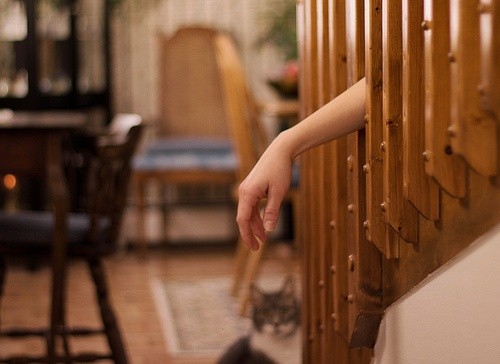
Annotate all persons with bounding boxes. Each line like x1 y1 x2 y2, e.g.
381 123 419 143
236 77 368 252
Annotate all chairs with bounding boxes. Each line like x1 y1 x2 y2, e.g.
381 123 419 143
1 112 144 364
124 26 257 248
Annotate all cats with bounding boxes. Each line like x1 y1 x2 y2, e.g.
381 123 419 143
217 272 302 364
